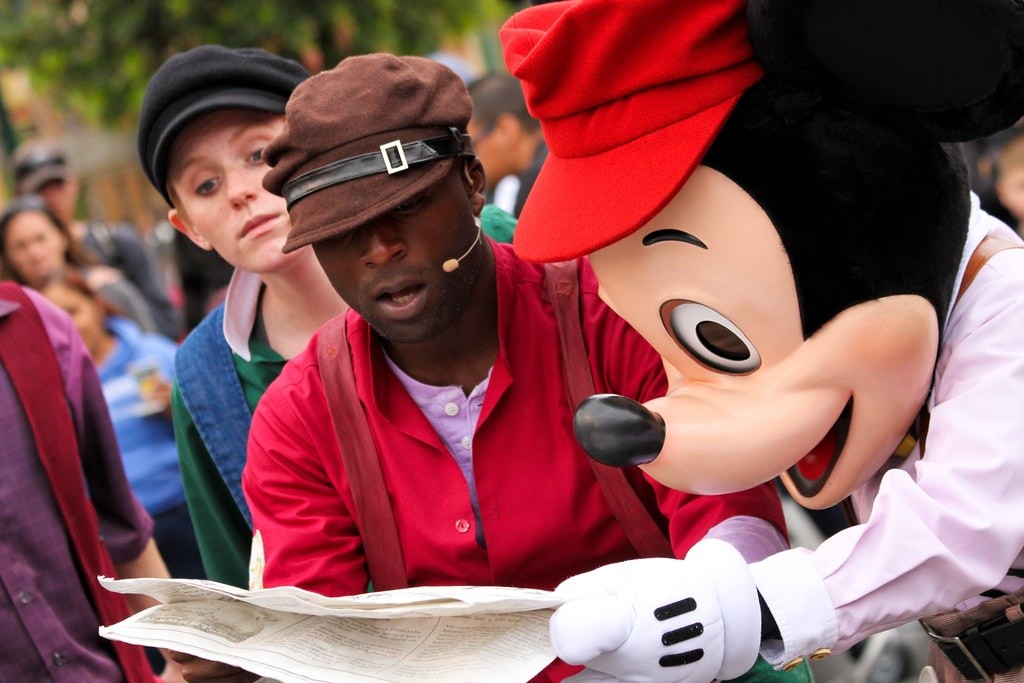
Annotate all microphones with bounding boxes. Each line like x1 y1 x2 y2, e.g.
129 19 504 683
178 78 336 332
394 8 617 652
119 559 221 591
442 228 481 273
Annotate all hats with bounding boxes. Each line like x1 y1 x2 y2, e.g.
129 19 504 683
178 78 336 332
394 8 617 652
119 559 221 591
137 43 313 208
262 52 476 253
14 145 70 194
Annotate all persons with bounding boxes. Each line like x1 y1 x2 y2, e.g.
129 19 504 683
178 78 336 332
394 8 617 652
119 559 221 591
0 44 1024 683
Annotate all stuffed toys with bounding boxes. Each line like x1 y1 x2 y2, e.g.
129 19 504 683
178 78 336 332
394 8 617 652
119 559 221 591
500 0 1024 683
